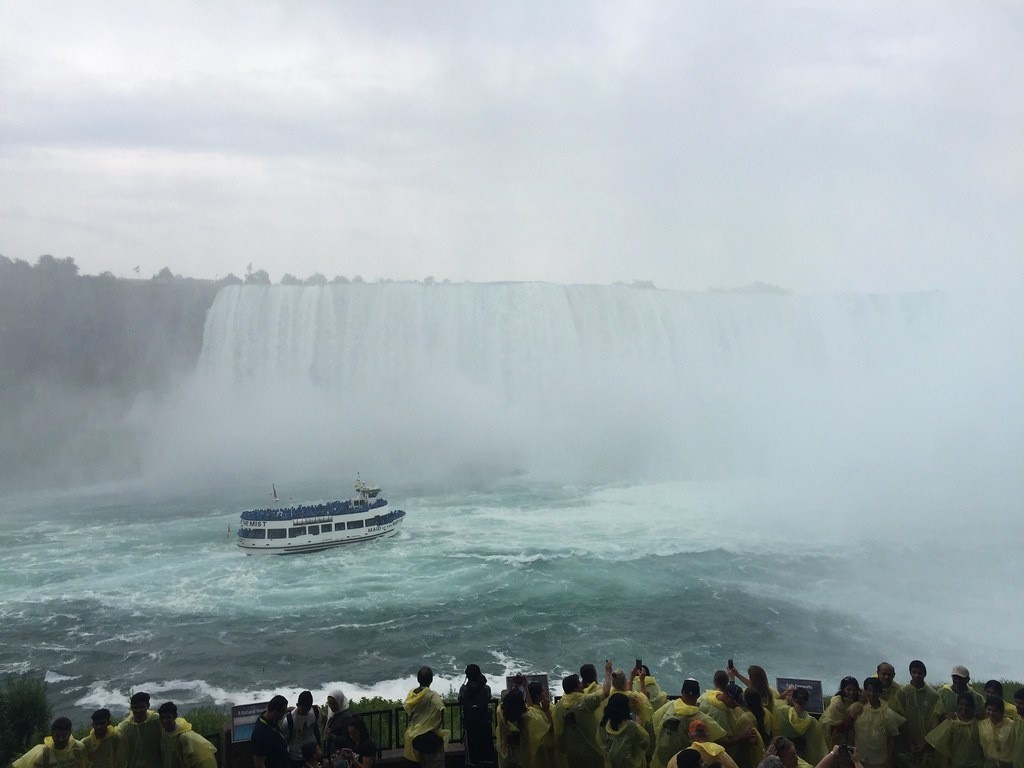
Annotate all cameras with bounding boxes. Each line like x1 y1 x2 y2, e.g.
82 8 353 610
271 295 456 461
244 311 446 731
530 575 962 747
513 675 524 686
839 744 854 757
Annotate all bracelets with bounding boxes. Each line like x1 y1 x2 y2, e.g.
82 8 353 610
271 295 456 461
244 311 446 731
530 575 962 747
830 751 835 756
730 680 735 681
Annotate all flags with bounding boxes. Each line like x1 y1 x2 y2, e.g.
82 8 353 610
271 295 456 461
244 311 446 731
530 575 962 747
272 484 279 502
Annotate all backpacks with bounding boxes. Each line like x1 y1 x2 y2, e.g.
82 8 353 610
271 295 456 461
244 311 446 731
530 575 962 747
287 706 321 744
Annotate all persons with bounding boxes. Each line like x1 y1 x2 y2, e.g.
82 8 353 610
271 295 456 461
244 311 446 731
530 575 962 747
237 497 406 539
11 659 1024 768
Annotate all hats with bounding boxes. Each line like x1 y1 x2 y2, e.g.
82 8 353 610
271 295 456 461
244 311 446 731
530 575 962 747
682 681 700 696
687 719 707 737
951 666 969 678
465 664 481 678
726 685 744 706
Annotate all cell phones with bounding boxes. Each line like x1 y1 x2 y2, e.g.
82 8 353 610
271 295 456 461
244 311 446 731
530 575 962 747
728 659 734 670
636 659 642 670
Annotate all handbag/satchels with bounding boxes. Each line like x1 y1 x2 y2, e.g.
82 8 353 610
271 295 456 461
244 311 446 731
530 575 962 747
412 730 444 753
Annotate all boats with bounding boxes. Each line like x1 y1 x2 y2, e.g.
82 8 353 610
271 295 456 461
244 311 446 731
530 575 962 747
236 480 406 556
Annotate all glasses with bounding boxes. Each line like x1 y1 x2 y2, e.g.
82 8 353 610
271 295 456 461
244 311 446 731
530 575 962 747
326 702 333 705
